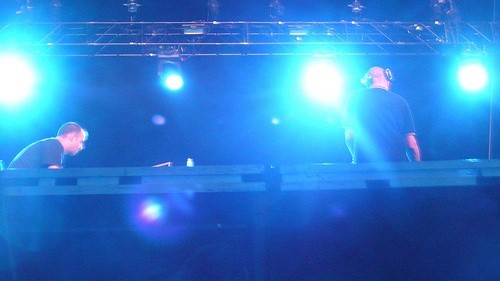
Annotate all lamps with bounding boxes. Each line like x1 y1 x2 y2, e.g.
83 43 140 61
157 58 186 94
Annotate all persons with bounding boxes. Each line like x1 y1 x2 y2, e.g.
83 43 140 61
344 66 422 161
6 121 88 170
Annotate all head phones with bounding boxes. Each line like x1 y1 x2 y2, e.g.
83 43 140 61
360 67 393 87
